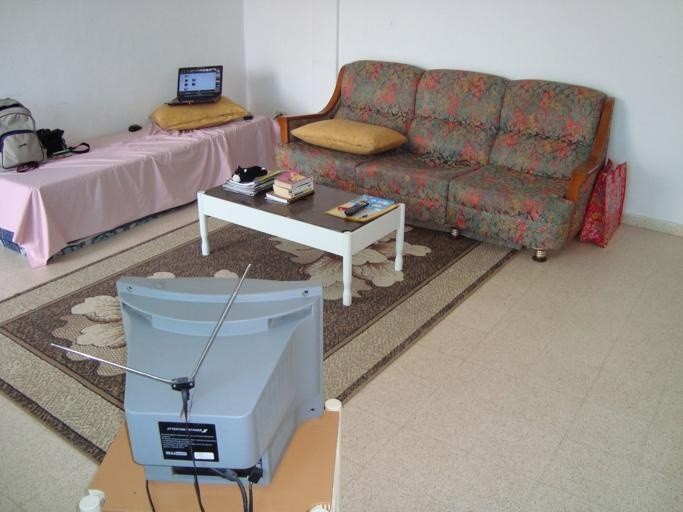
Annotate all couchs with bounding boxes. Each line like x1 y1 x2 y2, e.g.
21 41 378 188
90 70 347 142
272 60 615 262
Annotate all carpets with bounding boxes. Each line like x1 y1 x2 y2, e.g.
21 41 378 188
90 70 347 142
0 215 524 467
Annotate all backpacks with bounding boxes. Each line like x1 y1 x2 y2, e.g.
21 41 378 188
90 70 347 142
0 97 48 169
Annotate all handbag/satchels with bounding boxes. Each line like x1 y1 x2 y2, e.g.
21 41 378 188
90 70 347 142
37 129 90 156
579 158 626 247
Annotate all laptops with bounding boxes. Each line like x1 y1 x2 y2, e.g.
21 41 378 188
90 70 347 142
168 65 223 105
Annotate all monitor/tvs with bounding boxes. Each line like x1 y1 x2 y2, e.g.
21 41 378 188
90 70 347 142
116 277 325 487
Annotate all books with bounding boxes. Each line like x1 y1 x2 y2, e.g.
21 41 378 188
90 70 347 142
223 166 314 204
327 194 399 222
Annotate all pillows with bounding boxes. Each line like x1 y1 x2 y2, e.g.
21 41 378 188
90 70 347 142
149 95 251 132
289 118 409 156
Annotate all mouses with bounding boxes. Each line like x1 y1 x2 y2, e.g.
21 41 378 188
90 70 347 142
129 124 142 132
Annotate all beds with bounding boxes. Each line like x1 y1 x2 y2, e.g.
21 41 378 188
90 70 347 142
0 114 272 265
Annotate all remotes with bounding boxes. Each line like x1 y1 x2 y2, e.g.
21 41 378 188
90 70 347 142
345 201 369 216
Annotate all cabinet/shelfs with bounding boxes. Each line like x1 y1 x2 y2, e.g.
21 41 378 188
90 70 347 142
77 398 342 512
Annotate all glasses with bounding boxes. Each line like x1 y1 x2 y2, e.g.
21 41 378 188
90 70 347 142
17 160 40 172
